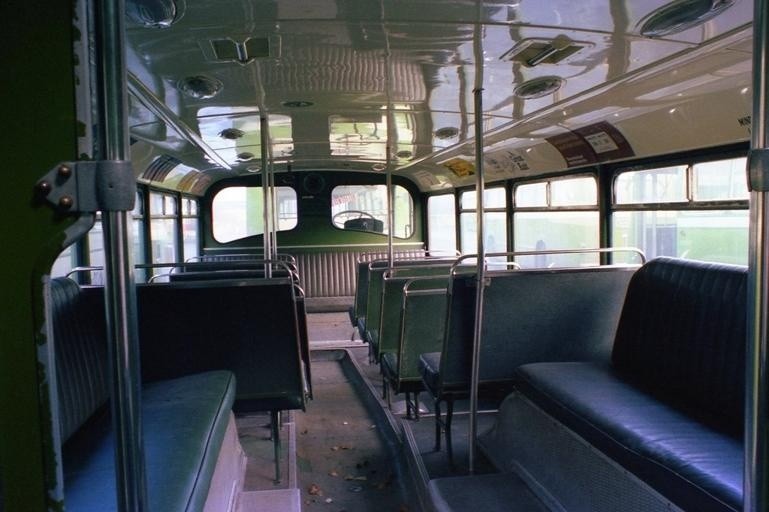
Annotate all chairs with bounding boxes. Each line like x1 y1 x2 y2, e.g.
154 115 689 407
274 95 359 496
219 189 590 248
382 276 450 422
360 257 488 341
49 276 238 512
65 261 310 484
293 283 313 400
370 263 522 375
418 246 647 470
516 255 751 511
169 262 298 273
343 219 382 232
348 250 461 342
186 254 296 265
147 269 301 287
